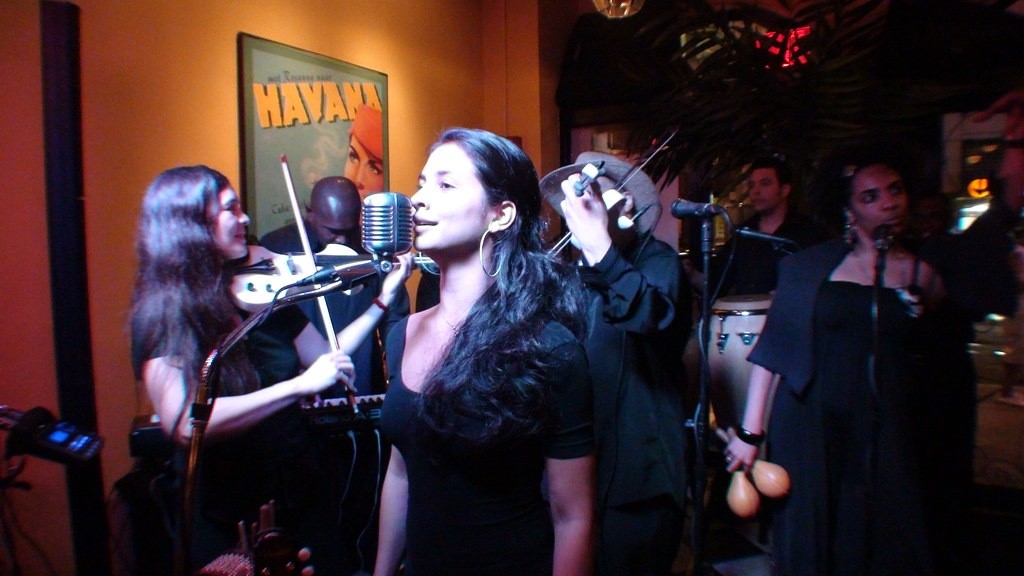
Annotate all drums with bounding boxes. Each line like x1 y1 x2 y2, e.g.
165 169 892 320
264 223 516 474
707 294 777 426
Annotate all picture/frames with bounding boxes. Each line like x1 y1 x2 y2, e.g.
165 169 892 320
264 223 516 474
235 31 390 245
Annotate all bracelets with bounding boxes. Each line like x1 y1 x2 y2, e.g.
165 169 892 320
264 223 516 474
734 425 766 447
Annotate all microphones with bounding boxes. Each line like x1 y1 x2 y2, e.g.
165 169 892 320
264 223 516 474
670 199 723 219
293 193 411 292
732 227 794 245
870 223 897 273
6 406 57 460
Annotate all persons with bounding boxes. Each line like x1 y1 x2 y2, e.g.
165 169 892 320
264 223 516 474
727 156 817 291
725 163 979 576
373 128 691 576
342 104 383 226
130 163 414 575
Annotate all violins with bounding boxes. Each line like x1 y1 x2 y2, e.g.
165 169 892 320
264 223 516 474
225 240 436 316
565 157 638 265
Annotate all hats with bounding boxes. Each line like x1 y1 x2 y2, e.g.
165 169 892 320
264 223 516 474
539 152 661 236
